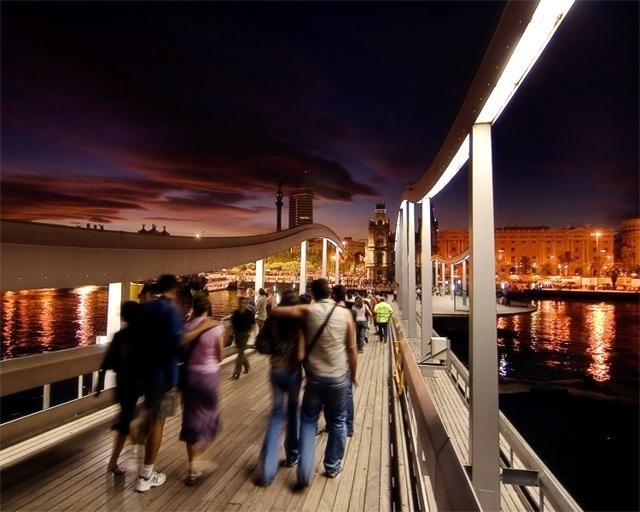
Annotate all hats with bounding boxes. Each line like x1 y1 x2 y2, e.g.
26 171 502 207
151 274 180 294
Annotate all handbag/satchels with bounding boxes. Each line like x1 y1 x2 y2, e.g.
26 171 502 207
99 322 136 372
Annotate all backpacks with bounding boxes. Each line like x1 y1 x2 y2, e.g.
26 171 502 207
252 313 286 356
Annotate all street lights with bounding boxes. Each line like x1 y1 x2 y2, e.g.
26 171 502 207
273 179 284 233
594 231 602 290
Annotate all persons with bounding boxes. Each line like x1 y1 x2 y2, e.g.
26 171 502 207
230 274 453 388
253 289 303 488
330 281 359 440
95 299 149 479
179 296 235 488
300 293 329 437
268 278 359 491
132 272 224 495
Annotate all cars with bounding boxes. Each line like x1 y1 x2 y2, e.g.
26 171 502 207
543 275 640 291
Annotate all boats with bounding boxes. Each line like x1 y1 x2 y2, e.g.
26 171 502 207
199 269 233 292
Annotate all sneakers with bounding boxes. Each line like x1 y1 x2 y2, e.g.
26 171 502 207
231 364 252 380
135 472 167 491
297 476 313 495
186 466 208 483
261 473 279 489
326 463 346 479
375 331 388 344
325 427 354 437
106 466 127 475
286 457 299 468
